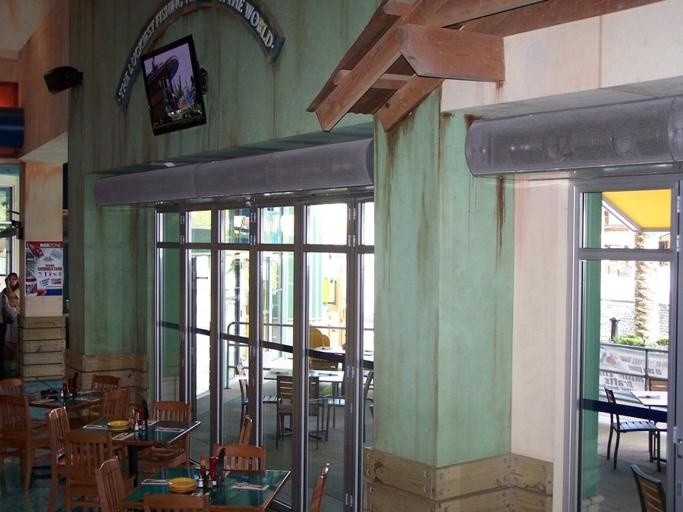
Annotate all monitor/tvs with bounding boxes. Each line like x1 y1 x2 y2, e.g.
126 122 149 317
139 35 206 136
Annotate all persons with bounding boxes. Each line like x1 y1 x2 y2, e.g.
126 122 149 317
0 273 21 378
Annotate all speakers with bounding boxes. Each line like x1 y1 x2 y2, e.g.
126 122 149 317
43 66 85 94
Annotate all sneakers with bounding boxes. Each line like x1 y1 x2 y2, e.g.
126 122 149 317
7 362 16 370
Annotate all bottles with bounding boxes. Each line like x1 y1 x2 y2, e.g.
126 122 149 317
196 448 226 488
59 376 78 399
128 406 147 432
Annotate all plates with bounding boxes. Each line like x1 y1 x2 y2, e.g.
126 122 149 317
106 420 129 427
167 477 197 492
107 427 128 432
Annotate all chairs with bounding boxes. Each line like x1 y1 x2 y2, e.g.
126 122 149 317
603 388 664 469
624 468 669 511
310 325 326 371
